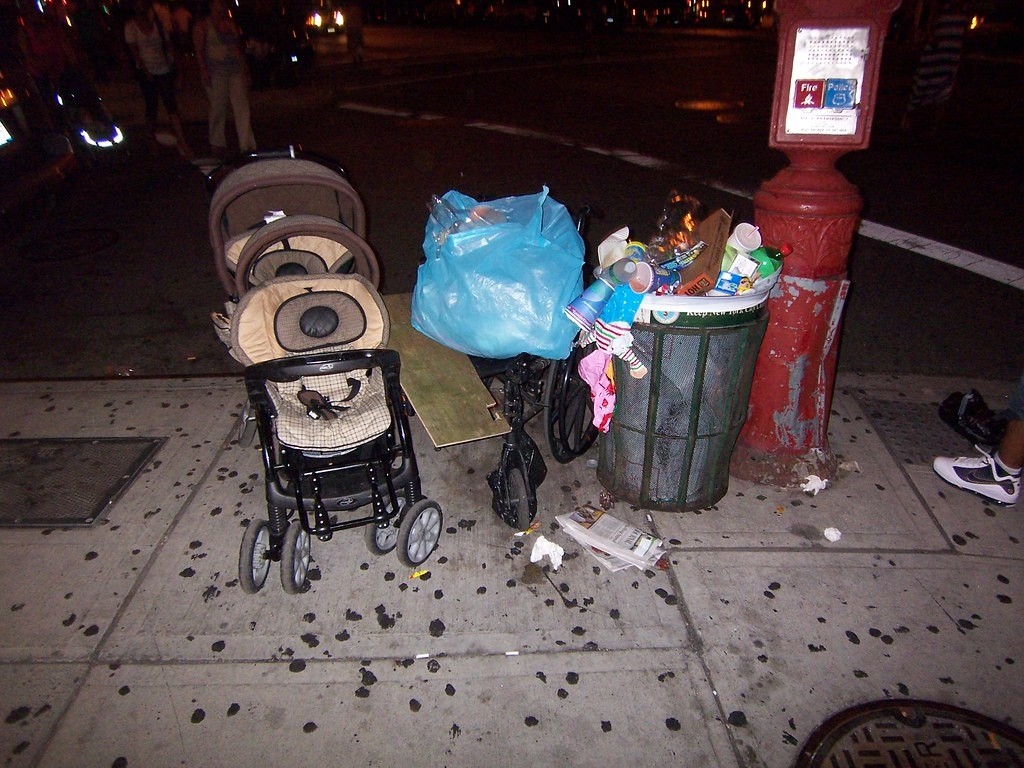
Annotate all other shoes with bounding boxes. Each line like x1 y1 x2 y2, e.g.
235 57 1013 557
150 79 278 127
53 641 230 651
212 146 229 158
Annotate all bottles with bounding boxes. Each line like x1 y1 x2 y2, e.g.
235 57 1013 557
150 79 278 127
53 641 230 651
600 259 635 285
747 244 792 282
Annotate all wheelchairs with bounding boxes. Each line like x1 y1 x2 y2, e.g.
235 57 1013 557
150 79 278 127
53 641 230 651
401 181 600 531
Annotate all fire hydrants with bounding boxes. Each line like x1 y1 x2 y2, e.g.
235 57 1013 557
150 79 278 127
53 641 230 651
727 0 897 485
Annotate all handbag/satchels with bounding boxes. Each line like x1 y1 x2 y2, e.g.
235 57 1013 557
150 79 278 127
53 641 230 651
243 46 266 80
160 73 184 108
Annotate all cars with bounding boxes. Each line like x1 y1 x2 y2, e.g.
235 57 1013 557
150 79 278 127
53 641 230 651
306 0 347 32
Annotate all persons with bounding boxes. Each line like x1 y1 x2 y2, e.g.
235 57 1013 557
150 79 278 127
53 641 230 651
933 377 1024 508
902 0 973 144
0 0 366 165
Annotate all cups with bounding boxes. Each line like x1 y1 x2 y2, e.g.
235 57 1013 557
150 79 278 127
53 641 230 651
564 278 614 332
625 242 645 265
728 223 763 252
628 262 682 293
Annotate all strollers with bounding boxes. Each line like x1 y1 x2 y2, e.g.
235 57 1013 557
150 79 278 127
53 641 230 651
203 146 443 595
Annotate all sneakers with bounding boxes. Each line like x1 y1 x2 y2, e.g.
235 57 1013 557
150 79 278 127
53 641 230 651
932 445 1020 507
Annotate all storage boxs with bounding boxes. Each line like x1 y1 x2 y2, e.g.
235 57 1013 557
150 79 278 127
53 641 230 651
676 207 735 295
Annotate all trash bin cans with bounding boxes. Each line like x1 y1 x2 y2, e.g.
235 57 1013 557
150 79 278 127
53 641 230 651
591 242 771 513
